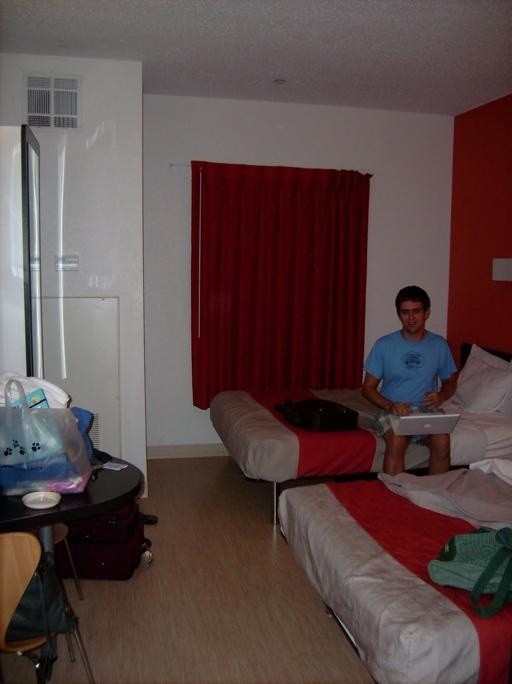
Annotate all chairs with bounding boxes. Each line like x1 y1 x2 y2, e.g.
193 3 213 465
27 522 84 600
0 531 96 684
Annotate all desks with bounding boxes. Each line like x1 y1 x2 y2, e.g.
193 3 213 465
0 456 141 660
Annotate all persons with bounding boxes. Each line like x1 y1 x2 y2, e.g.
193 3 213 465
363 283 464 475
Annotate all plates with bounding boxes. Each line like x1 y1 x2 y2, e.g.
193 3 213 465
21 492 63 510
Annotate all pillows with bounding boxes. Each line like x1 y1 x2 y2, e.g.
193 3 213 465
451 343 512 414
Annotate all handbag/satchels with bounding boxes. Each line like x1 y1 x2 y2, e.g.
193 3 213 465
0 379 93 497
277 397 360 430
427 526 511 617
6 549 79 646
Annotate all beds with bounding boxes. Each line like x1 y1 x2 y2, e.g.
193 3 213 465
209 389 512 526
278 468 512 684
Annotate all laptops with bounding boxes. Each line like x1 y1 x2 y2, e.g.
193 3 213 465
388 412 460 436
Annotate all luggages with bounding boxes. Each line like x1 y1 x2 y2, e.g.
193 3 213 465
60 502 161 580
